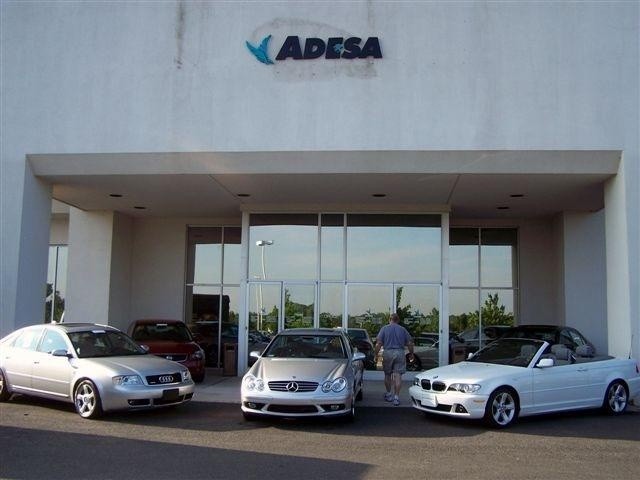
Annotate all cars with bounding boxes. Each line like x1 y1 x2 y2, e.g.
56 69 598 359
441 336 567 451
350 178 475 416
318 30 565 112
373 326 509 371
341 329 373 369
192 321 271 366
497 325 599 358
128 319 205 383
240 328 366 421
408 338 639 426
1 323 195 419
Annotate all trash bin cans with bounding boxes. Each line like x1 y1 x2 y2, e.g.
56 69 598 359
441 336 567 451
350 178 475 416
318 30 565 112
451 342 467 364
224 342 238 377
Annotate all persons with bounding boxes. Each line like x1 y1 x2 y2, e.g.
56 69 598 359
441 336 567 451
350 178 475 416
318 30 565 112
373 313 414 406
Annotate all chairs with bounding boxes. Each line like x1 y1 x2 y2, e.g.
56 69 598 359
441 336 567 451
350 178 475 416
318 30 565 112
318 343 339 357
520 345 536 359
575 344 592 361
555 349 572 365
550 343 566 354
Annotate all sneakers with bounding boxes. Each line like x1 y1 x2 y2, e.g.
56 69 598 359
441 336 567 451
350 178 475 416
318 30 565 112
384 393 399 405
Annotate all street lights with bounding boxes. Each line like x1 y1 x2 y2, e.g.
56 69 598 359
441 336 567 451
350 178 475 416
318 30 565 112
255 274 270 332
256 239 274 281
